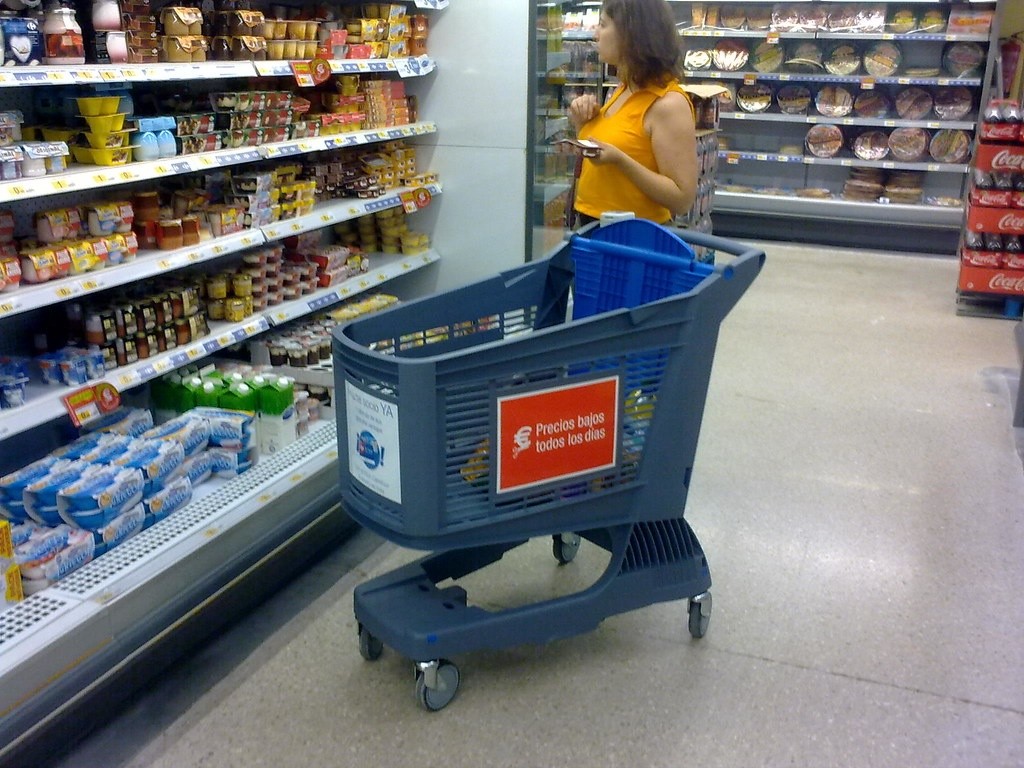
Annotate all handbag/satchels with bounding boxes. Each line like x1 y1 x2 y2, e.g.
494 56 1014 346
565 179 576 232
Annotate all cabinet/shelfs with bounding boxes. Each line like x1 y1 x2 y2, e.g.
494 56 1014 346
536 0 1024 321
0 0 443 768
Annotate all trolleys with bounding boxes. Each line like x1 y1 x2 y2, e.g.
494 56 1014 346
329 212 767 713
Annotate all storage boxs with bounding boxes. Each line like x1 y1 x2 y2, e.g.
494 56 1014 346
977 144 1024 172
980 121 1020 142
969 188 1012 207
1001 255 1024 270
1010 191 1024 209
962 246 1000 267
959 267 1023 294
298 79 440 203
967 192 1024 234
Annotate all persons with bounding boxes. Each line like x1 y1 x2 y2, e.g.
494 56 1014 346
570 0 697 227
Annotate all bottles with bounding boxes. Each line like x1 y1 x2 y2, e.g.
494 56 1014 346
622 389 657 463
956 99 1024 298
43 9 86 65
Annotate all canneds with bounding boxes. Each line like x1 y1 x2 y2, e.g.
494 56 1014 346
0 192 245 294
83 282 212 371
161 7 267 61
